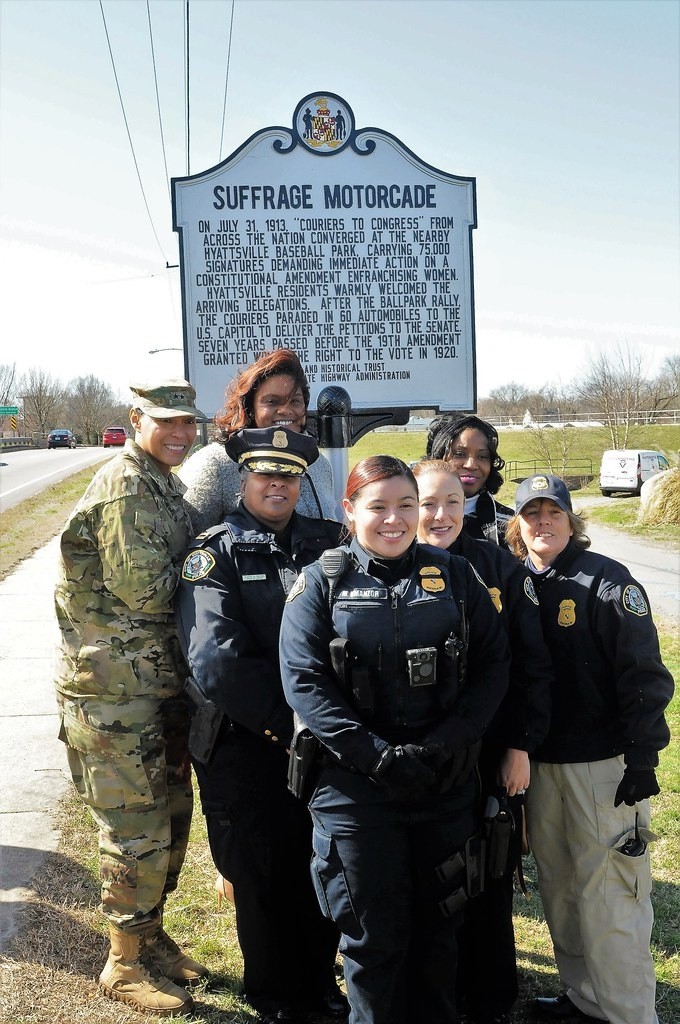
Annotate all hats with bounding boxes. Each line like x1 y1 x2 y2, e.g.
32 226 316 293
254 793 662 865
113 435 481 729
225 426 320 477
130 376 207 419
514 473 572 515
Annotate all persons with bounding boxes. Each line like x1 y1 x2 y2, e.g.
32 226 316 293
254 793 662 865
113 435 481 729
412 460 519 588
172 425 353 1024
278 456 555 1024
500 474 676 1024
425 412 518 550
176 352 338 909
55 375 206 1013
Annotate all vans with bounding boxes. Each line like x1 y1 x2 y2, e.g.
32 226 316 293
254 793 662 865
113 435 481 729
600 450 670 498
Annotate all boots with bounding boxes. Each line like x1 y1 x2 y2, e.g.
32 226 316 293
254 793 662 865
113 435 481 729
138 897 208 986
98 924 193 1018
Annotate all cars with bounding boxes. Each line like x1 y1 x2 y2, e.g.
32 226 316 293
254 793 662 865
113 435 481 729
47 430 77 449
103 427 126 448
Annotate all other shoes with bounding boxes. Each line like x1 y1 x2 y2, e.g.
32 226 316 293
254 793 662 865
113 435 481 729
296 969 349 1019
527 993 610 1024
246 990 308 1024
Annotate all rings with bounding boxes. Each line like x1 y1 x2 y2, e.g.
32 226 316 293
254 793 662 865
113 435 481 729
517 789 525 793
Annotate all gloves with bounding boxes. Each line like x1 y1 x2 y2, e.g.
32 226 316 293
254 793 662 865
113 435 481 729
614 768 660 808
372 741 446 807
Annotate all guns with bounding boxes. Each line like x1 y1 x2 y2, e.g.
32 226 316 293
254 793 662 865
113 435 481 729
182 676 225 727
